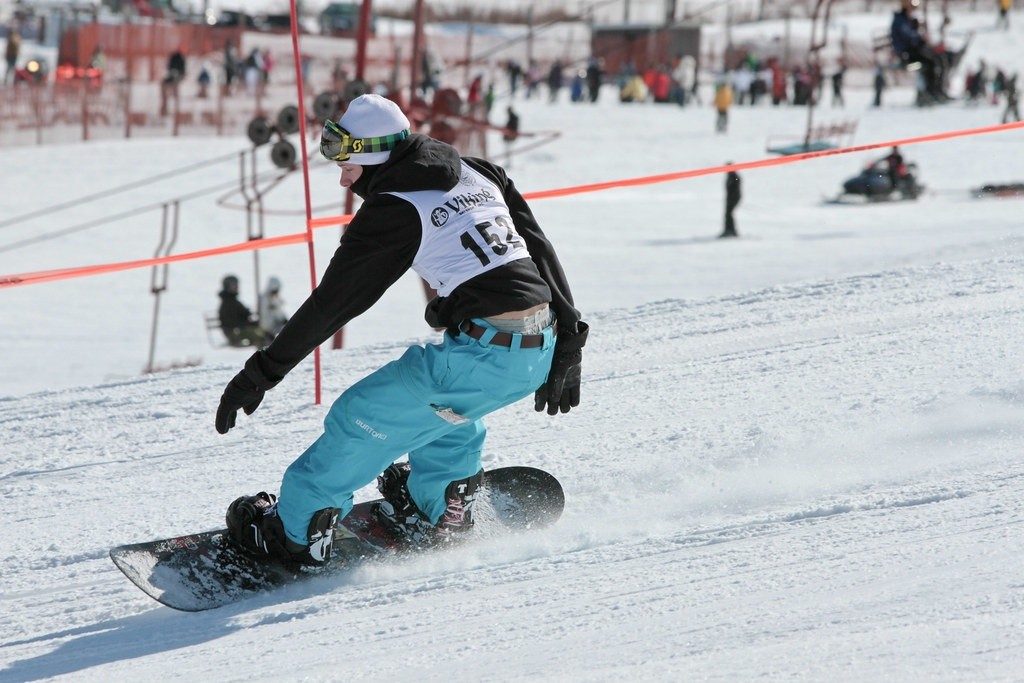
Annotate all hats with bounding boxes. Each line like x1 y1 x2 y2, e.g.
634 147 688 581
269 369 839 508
268 278 280 291
338 93 411 165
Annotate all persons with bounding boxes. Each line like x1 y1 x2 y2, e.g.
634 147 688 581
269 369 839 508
2 24 23 83
502 106 522 167
255 277 291 341
866 144 904 191
218 274 275 348
892 0 1023 127
215 93 589 569
85 33 563 123
717 162 742 237
571 39 891 133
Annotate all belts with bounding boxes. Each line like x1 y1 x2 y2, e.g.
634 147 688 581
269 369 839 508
454 316 558 349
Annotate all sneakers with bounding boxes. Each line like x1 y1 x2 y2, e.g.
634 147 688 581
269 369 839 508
378 461 475 540
225 496 333 572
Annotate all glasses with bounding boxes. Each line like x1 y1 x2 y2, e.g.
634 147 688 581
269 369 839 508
319 119 411 161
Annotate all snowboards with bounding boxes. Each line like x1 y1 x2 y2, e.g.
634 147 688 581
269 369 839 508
112 466 565 613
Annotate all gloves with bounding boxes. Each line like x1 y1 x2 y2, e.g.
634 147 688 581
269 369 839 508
215 348 284 434
534 320 590 415
